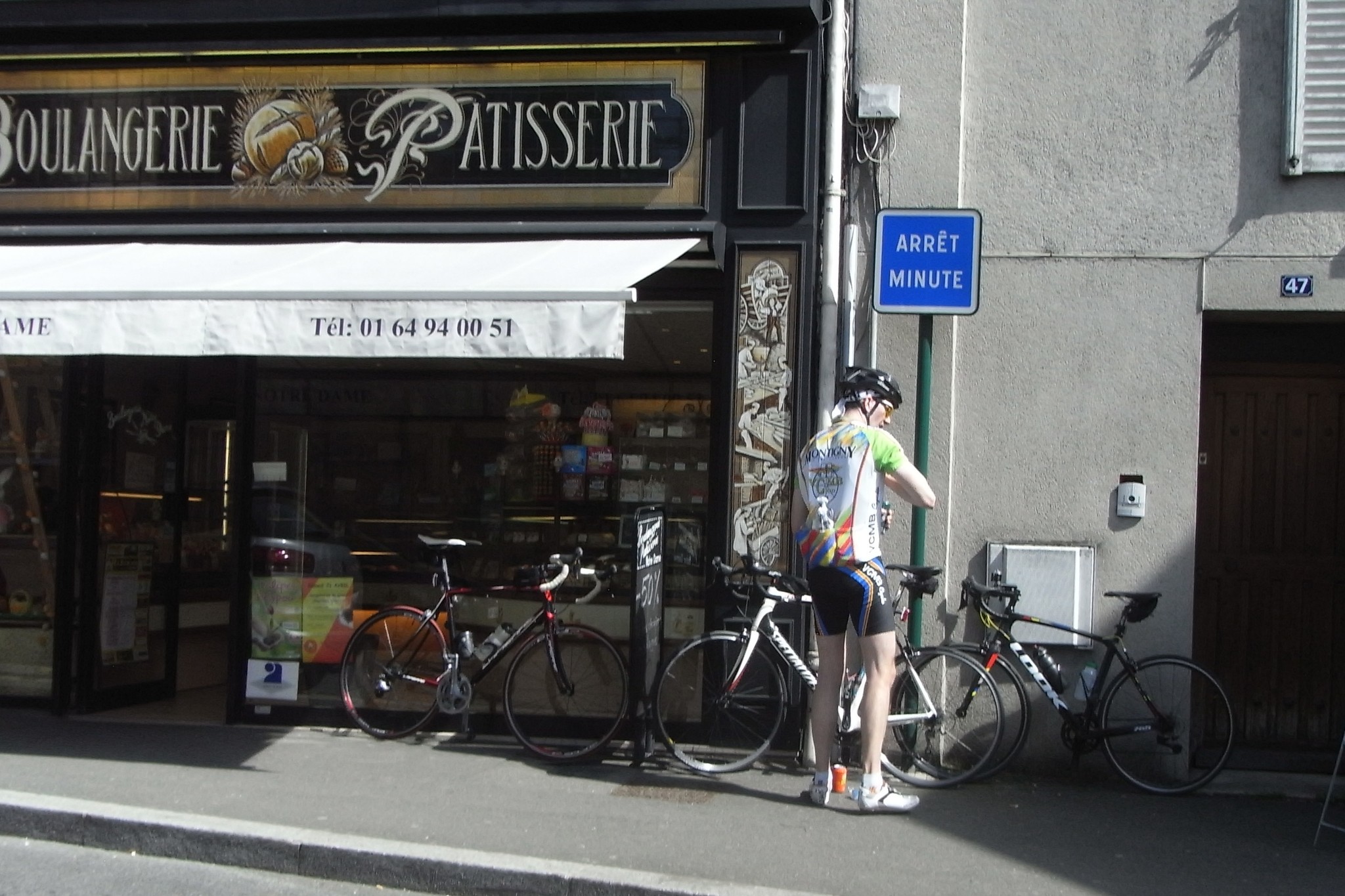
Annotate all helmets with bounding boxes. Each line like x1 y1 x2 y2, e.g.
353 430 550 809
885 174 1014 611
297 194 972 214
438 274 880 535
839 366 903 410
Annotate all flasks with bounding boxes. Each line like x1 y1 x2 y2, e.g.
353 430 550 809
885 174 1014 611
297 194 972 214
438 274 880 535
457 630 474 659
1032 643 1070 693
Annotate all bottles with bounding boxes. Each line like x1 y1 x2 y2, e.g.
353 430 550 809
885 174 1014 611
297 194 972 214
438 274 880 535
1073 661 1097 701
474 623 511 660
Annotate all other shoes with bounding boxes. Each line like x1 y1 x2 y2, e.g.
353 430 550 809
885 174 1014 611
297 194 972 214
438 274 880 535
857 782 919 813
808 777 833 805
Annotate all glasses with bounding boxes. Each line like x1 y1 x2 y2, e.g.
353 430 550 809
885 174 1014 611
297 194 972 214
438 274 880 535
883 403 893 417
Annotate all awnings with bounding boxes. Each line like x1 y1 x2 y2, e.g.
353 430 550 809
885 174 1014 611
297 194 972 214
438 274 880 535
0 219 727 361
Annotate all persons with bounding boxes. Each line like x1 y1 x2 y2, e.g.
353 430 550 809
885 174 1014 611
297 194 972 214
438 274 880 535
792 365 936 811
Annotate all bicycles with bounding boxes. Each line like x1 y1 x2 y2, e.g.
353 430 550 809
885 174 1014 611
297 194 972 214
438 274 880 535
650 555 1004 791
891 572 1234 797
341 532 629 764
